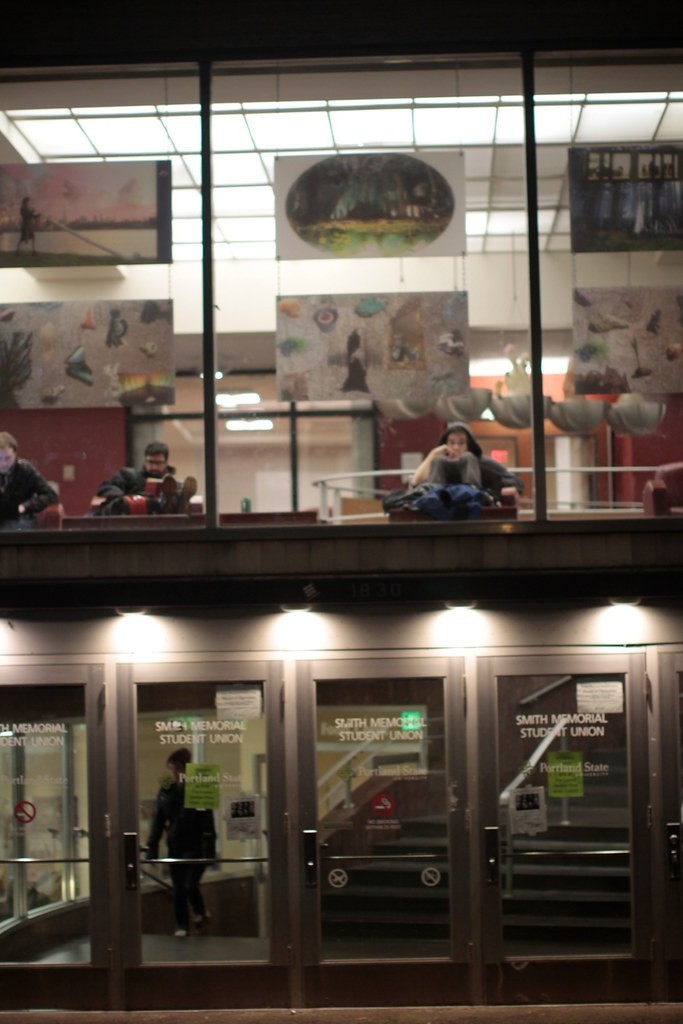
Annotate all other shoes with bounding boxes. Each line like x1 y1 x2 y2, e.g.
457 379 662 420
158 473 179 514
174 473 198 514
173 927 185 938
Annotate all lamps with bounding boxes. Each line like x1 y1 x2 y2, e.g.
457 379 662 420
372 299 667 435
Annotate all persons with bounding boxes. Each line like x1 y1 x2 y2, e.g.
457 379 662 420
145 749 217 940
91 442 193 515
0 432 59 530
408 422 524 506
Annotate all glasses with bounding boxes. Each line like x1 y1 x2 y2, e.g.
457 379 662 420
146 459 168 465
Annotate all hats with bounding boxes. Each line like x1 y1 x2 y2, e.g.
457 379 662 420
166 747 194 773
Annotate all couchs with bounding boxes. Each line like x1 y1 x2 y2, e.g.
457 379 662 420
642 460 683 518
32 503 67 532
88 495 205 516
388 485 519 522
